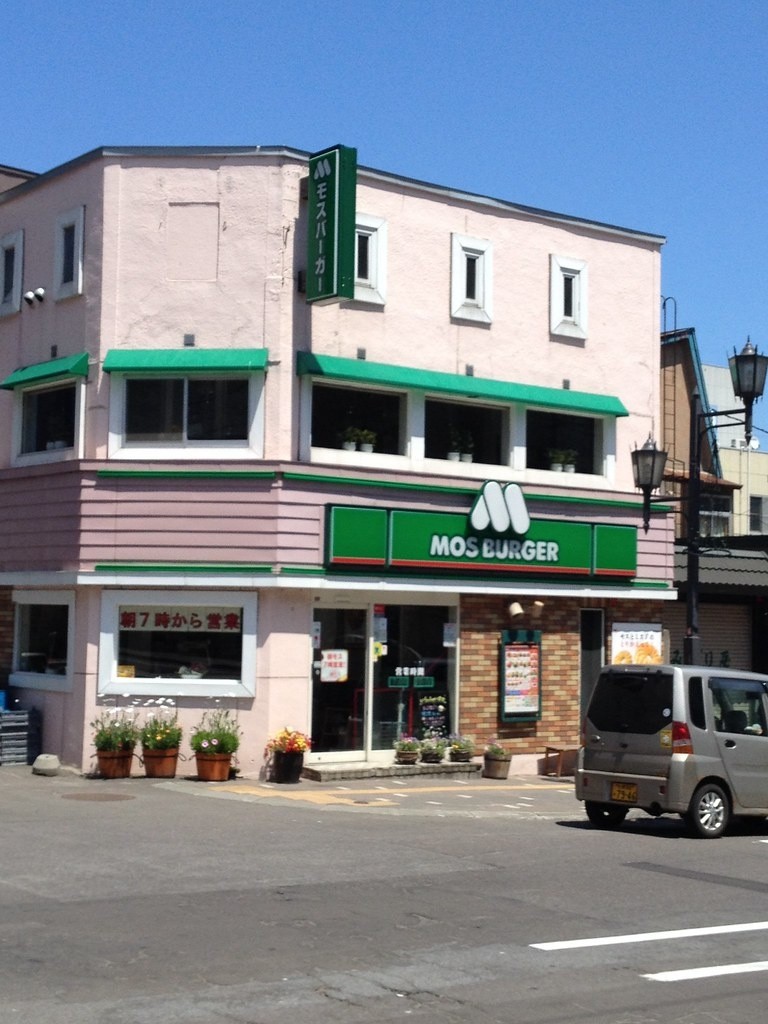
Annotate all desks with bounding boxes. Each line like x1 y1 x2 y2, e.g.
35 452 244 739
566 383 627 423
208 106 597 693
541 744 582 777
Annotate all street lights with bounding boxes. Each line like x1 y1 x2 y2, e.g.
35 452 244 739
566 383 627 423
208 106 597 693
631 334 767 665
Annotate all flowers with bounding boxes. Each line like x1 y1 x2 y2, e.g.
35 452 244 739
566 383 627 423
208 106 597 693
393 733 475 759
190 699 243 756
263 727 315 759
483 738 509 754
90 692 183 753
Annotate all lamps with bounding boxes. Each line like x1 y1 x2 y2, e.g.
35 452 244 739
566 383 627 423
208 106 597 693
505 600 544 621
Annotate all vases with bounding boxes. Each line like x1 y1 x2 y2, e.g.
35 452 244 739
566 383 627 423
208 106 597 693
142 748 179 777
196 752 231 781
421 751 441 762
484 755 512 779
397 750 418 764
97 750 133 778
450 751 469 762
273 751 303 784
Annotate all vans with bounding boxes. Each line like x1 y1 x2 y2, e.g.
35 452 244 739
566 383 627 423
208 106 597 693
574 662 768 840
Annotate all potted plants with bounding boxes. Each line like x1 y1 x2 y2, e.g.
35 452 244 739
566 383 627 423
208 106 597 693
46 427 70 450
340 426 377 452
445 433 474 463
549 448 579 472
